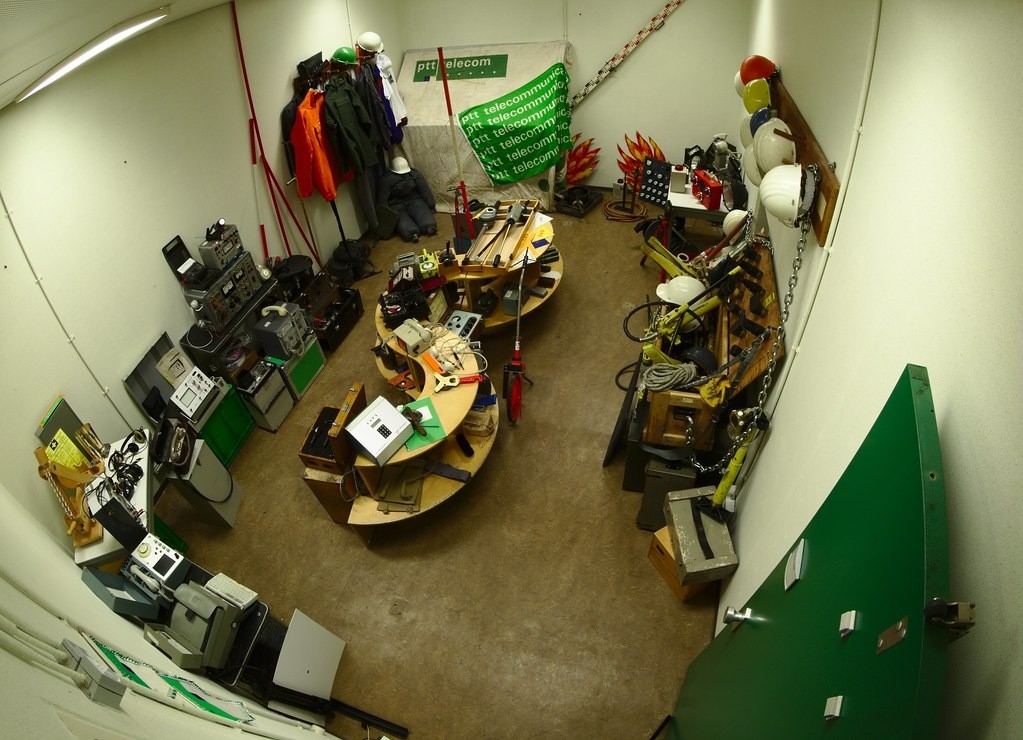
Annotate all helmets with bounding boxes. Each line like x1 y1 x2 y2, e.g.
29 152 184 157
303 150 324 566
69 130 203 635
739 114 754 148
757 164 803 227
330 47 359 65
732 71 745 96
723 210 749 245
388 157 412 174
357 31 385 53
754 117 796 172
749 106 771 137
722 178 748 212
742 142 767 187
655 277 707 311
684 345 720 376
741 78 771 113
740 55 775 85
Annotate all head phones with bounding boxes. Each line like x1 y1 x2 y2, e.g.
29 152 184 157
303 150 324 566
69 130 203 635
120 429 145 455
113 463 143 500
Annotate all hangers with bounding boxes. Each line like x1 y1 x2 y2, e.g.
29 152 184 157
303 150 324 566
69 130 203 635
290 49 379 92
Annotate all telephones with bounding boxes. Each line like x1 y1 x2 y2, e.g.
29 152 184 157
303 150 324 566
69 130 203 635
261 305 289 318
190 300 206 330
170 430 186 463
119 532 192 610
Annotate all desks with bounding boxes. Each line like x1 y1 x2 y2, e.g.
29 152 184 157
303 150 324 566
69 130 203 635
304 469 352 521
180 279 278 385
276 254 313 301
74 427 151 567
665 176 744 272
115 552 282 703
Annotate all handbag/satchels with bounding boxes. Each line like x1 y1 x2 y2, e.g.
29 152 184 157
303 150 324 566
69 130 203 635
332 239 372 265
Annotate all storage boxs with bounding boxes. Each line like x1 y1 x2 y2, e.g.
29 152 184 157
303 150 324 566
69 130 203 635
648 484 739 604
298 380 366 474
300 272 366 353
199 384 256 468
264 329 328 400
642 237 784 452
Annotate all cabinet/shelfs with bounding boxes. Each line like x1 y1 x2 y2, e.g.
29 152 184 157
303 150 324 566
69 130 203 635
347 199 560 529
